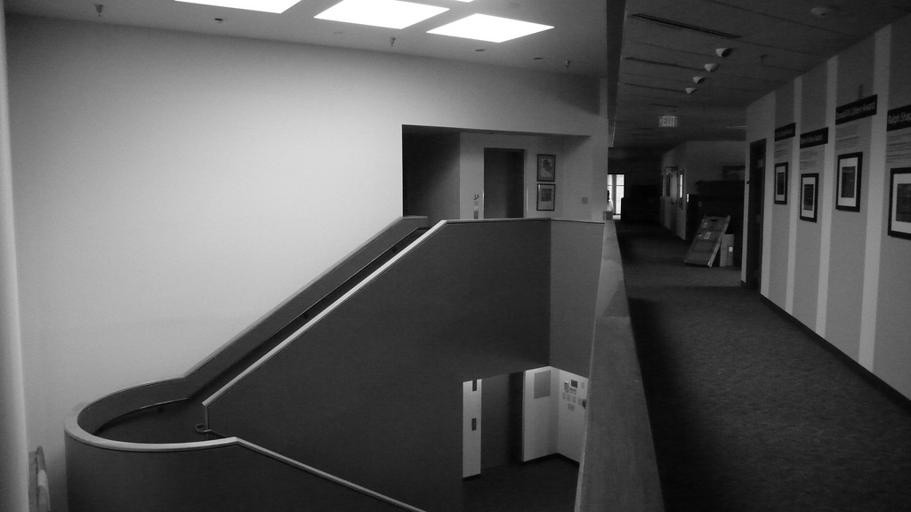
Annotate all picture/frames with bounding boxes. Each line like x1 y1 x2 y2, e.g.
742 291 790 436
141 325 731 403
888 166 911 241
537 154 555 181
774 162 788 204
836 152 863 212
536 183 555 211
800 173 817 223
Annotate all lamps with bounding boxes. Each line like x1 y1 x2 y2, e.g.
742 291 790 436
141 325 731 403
685 48 732 97
